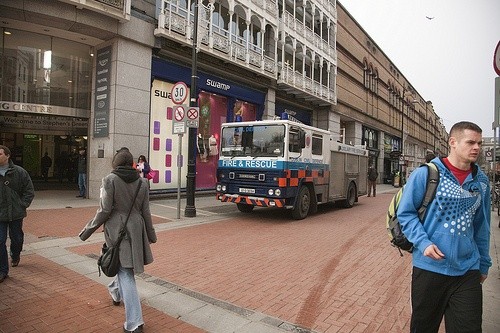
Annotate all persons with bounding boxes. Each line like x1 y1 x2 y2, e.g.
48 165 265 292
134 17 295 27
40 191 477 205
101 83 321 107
136 155 151 188
396 122 491 332
366 163 378 198
78 150 158 333
392 169 400 177
42 153 52 182
77 147 86 197
0 145 35 282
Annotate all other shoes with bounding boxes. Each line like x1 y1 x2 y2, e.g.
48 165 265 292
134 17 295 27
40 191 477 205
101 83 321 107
76 194 86 199
0 270 9 283
10 255 20 267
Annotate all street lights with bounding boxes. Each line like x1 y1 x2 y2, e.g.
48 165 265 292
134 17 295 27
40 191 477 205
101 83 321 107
399 100 420 189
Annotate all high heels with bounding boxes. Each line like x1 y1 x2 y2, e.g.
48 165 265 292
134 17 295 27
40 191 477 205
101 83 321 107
109 292 120 305
123 325 143 333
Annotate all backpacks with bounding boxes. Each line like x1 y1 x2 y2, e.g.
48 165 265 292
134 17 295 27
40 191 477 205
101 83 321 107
368 169 378 181
385 163 440 257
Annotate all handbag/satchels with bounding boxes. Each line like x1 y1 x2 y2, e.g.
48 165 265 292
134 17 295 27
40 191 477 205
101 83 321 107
144 167 156 179
97 243 120 278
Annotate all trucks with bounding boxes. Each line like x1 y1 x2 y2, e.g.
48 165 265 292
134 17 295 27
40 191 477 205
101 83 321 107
211 115 370 219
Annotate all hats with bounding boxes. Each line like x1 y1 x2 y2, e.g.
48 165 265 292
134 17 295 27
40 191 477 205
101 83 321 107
78 146 85 150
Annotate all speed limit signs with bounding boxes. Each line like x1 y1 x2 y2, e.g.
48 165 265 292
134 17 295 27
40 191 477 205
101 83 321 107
170 82 188 105
